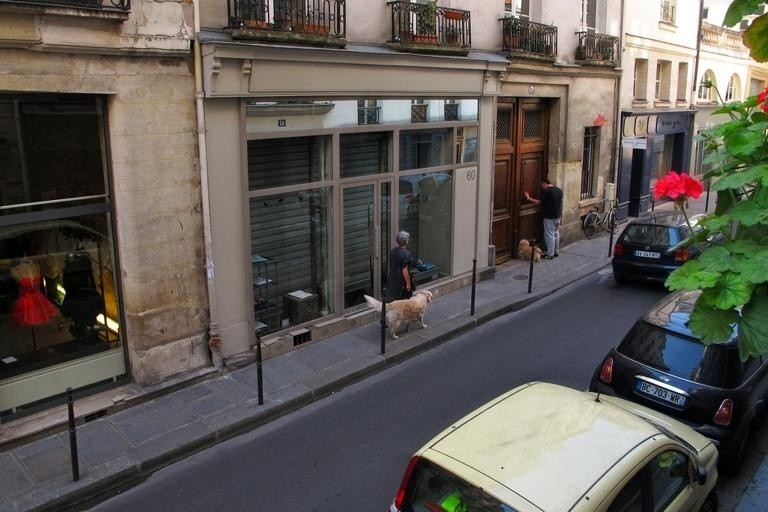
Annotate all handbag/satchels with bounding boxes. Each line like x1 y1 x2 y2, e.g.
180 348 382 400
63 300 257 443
402 274 416 299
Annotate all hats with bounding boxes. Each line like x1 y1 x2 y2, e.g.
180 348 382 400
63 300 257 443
397 231 410 241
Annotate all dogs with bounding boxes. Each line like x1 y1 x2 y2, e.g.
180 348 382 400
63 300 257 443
363 288 433 339
518 239 542 263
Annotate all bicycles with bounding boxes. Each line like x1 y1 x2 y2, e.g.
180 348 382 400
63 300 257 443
581 198 621 241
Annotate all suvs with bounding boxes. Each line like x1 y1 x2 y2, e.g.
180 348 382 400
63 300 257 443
612 208 726 290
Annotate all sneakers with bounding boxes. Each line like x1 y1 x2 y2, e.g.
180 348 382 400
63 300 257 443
540 253 559 259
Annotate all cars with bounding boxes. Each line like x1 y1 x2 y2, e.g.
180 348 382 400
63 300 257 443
390 377 721 512
589 288 767 473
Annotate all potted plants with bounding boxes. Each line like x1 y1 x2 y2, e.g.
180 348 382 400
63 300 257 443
439 24 463 45
403 0 438 45
273 0 293 31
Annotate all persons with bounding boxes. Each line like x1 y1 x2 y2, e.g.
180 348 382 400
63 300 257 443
11 257 58 325
524 177 563 259
388 231 412 302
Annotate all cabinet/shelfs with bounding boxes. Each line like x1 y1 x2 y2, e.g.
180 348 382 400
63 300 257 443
252 256 281 339
